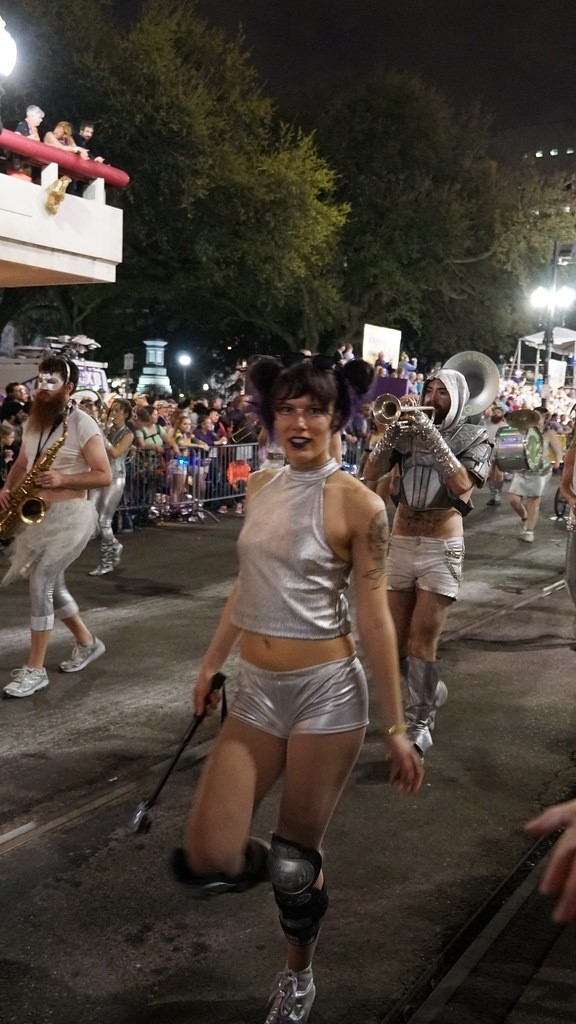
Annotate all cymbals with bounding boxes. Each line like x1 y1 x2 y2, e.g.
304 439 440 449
505 409 540 428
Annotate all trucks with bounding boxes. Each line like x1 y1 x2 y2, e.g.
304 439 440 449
0 336 109 404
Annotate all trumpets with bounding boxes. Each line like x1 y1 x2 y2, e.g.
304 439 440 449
373 393 438 432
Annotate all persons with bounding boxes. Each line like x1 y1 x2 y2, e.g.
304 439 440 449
560 421 576 606
88 398 135 574
363 368 495 758
525 799 576 919
508 408 561 542
166 351 422 1024
0 344 576 533
4 104 112 198
0 356 111 695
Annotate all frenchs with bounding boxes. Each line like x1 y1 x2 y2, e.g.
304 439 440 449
441 348 500 427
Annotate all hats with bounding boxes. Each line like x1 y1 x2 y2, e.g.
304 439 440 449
155 400 172 408
133 392 148 400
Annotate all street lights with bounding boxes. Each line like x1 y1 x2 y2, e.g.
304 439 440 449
528 239 576 408
179 355 191 397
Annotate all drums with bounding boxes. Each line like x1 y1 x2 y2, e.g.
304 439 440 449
494 426 544 472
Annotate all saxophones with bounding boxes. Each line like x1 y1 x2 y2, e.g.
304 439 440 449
0 411 69 540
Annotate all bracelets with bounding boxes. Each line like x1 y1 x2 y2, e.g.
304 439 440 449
382 724 410 736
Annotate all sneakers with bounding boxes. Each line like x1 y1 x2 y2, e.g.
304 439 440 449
3 665 50 697
263 960 316 1024
60 634 105 672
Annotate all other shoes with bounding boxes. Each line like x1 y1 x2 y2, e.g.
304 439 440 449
235 503 243 513
114 543 124 567
88 566 114 576
217 505 228 514
519 517 527 539
487 494 502 505
523 530 535 543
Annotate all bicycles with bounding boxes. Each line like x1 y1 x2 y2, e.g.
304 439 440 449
554 482 573 517
